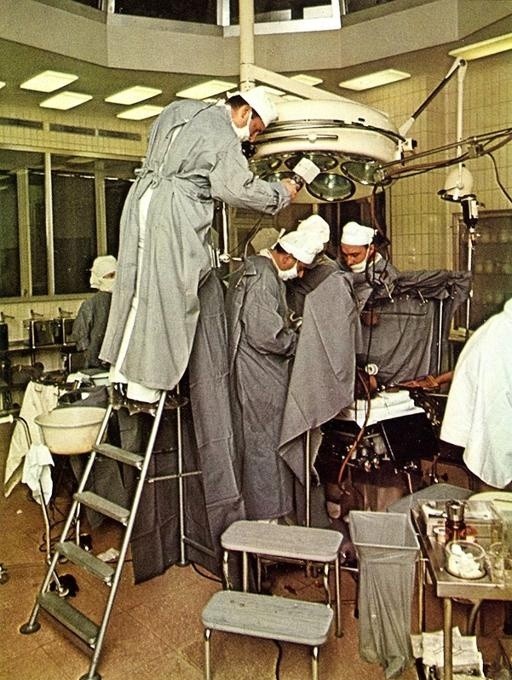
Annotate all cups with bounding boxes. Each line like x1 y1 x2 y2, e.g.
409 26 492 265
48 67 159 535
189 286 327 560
445 541 486 604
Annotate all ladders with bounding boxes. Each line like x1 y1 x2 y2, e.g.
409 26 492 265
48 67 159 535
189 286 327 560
20 391 217 680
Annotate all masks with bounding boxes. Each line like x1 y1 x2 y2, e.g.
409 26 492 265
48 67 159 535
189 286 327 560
232 119 251 141
349 258 368 274
277 262 298 281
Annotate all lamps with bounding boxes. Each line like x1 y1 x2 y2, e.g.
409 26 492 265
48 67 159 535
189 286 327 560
238 0 475 204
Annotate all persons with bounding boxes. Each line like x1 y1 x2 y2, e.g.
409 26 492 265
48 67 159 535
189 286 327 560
335 221 397 287
285 214 340 332
223 231 318 524
70 255 118 372
98 86 300 413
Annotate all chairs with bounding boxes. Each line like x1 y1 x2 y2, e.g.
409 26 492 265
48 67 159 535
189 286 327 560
201 520 344 680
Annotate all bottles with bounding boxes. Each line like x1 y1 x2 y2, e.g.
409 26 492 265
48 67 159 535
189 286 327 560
444 500 467 546
463 194 478 233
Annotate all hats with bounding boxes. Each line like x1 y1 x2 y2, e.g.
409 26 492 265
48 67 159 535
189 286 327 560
280 211 374 265
91 255 116 278
240 87 278 126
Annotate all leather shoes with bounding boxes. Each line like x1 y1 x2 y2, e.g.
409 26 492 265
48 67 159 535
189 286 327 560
127 391 189 410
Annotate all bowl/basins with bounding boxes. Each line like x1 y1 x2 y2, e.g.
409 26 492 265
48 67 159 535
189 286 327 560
34 406 107 455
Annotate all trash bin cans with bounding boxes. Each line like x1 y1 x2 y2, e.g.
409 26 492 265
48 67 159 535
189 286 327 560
349 510 420 666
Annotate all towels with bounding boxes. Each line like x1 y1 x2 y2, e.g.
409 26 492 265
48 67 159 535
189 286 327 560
21 444 55 505
